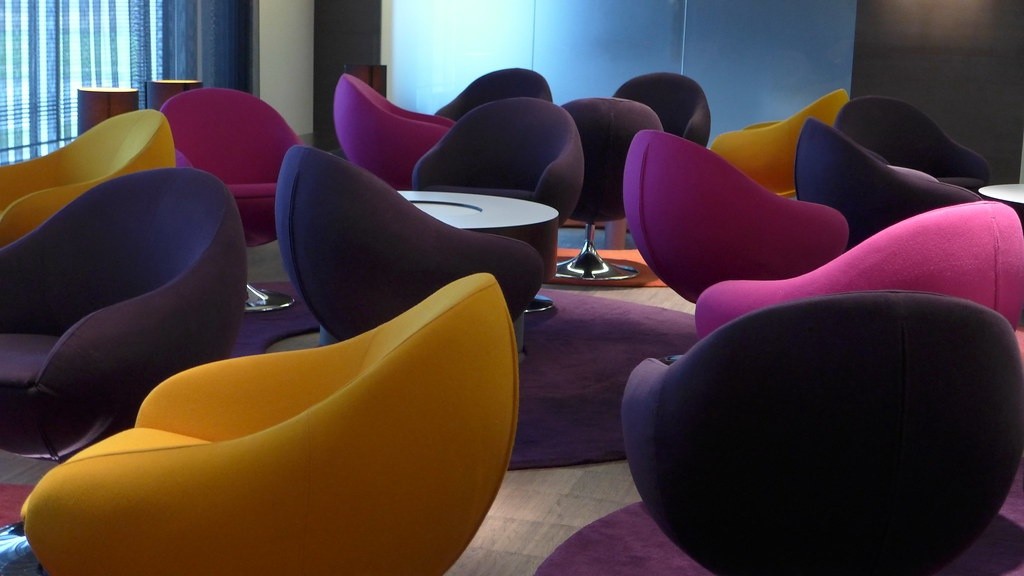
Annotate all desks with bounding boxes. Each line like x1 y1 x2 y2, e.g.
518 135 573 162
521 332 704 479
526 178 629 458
978 184 1024 238
396 190 559 362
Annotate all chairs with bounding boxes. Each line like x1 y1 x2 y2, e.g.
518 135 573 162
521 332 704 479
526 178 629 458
622 130 850 365
434 68 553 121
622 290 1024 576
333 74 456 190
794 117 982 251
275 145 545 341
412 97 584 226
556 97 664 280
710 88 849 199
21 272 519 576
0 168 246 462
0 109 176 249
696 200 1024 343
611 72 711 147
833 95 992 193
160 88 305 312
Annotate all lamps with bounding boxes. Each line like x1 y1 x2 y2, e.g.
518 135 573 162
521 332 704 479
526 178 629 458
77 86 138 138
344 64 387 99
148 80 204 111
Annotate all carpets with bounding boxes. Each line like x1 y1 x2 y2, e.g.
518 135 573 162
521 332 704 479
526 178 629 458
545 248 670 288
230 282 697 472
534 456 1024 576
